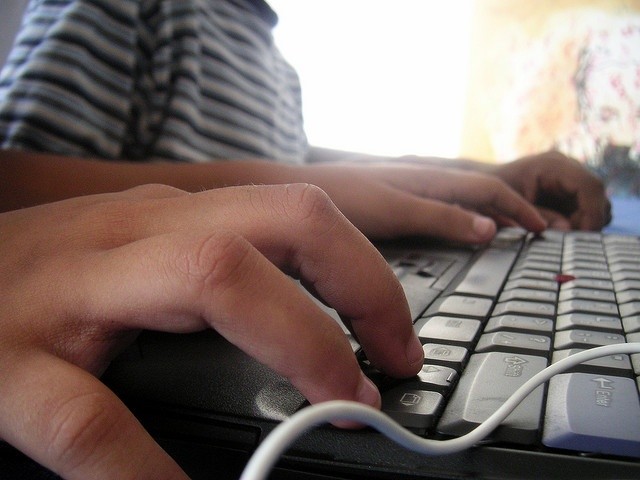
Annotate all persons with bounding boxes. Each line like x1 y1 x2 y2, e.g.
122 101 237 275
0 1 612 232
0 149 549 479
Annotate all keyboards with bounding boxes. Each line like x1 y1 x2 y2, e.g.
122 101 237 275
100 227 639 478
